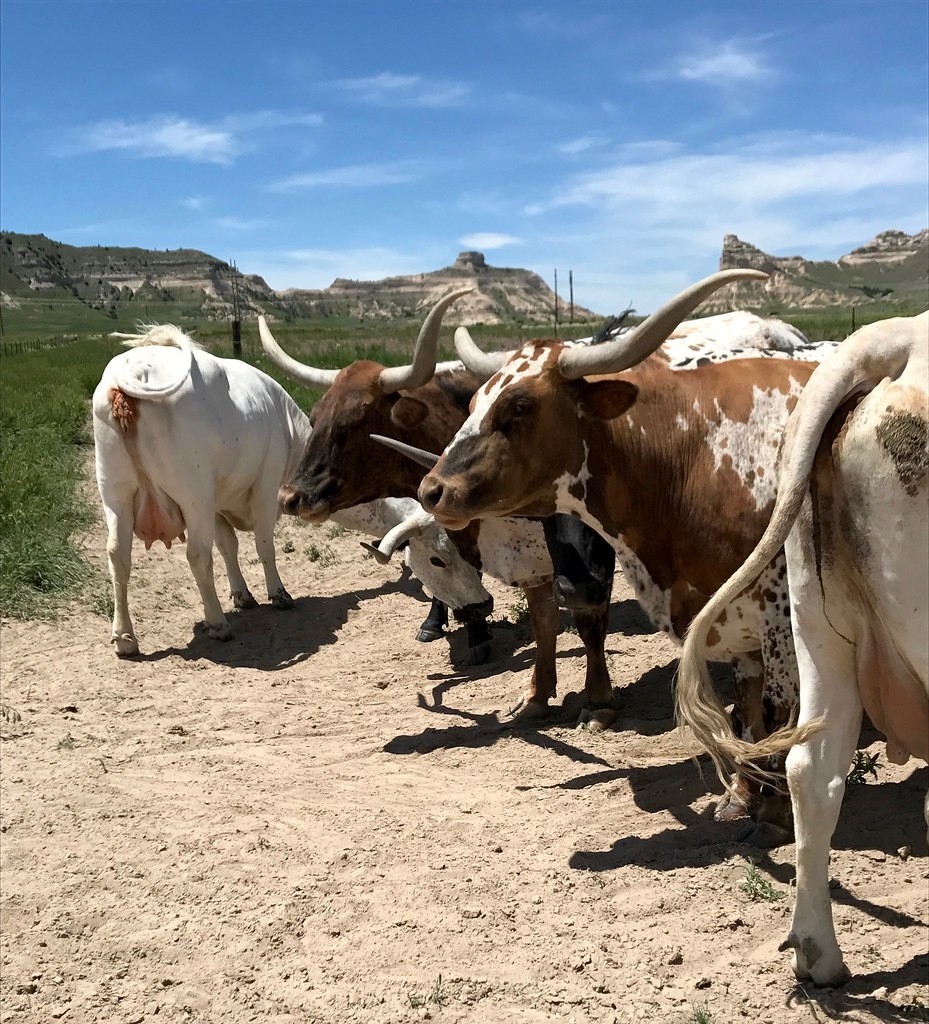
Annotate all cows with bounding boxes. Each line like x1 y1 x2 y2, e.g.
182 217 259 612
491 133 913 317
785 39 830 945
92 269 929 990
